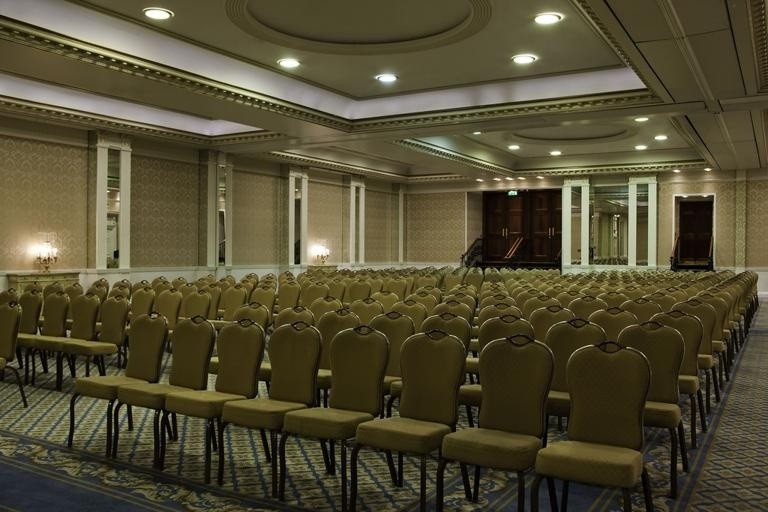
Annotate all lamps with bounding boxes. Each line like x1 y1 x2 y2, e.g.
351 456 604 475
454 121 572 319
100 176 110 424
35 241 58 270
312 245 329 262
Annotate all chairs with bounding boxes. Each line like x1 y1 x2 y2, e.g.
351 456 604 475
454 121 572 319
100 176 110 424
530 343 650 511
3 266 757 445
348 333 471 512
279 324 395 511
218 321 328 496
619 322 692 500
435 335 559 509
161 312 273 485
